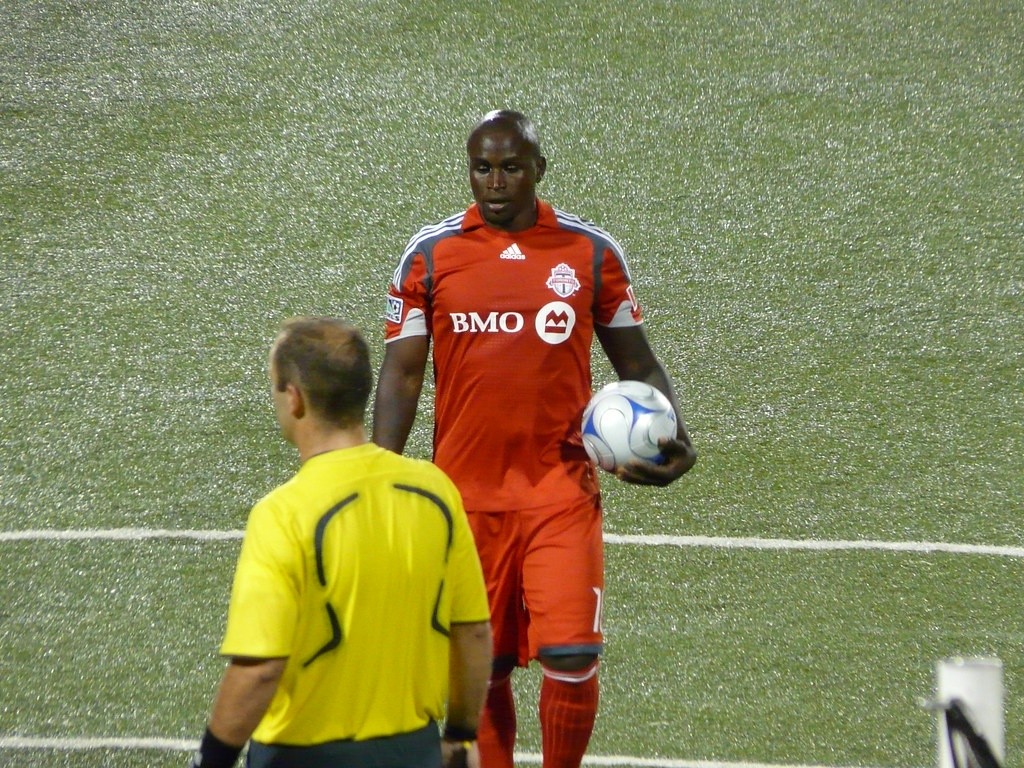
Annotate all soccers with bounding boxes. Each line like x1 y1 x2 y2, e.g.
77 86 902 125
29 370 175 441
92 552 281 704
582 380 678 472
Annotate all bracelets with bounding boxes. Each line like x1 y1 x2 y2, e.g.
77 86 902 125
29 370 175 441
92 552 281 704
194 727 244 768
445 726 477 742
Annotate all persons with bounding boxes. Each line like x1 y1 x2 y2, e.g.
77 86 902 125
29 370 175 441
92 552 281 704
194 317 494 768
373 109 697 768
